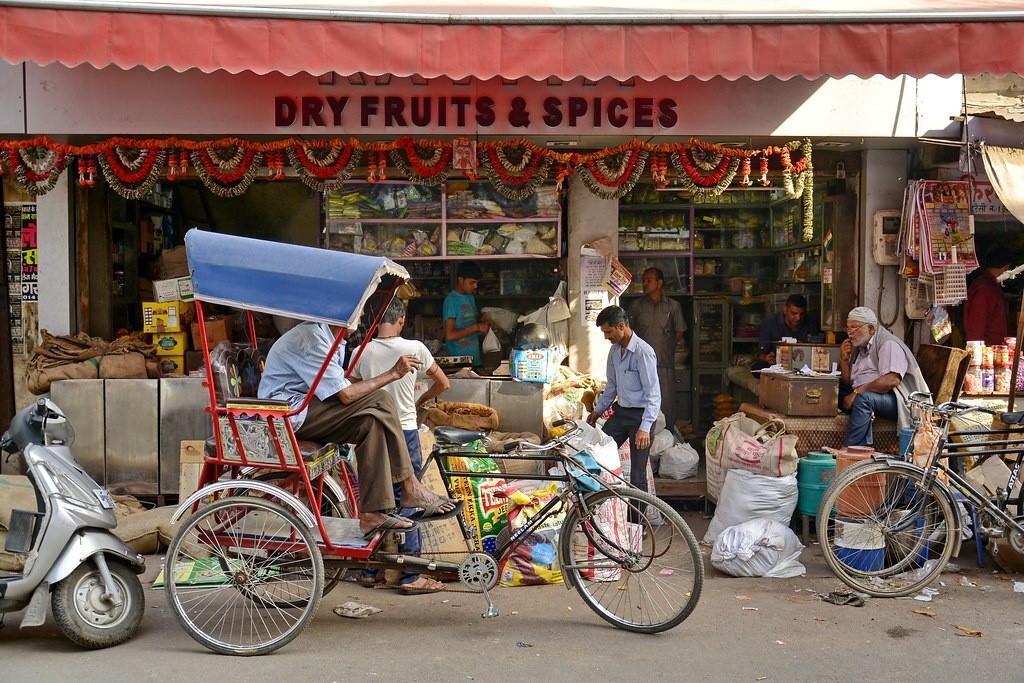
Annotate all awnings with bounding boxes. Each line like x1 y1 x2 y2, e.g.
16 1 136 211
980 145 1024 226
0 0 1024 83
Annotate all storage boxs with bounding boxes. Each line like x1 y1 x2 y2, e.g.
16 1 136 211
627 519 645 554
754 366 842 419
139 244 236 379
947 395 1024 520
508 343 566 384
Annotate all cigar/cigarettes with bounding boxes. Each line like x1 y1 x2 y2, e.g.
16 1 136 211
412 353 416 360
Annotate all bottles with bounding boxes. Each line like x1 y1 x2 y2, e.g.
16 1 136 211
899 423 937 458
836 446 886 516
799 452 836 517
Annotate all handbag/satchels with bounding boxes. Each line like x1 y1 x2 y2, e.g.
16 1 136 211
563 450 601 492
705 412 799 499
483 328 502 354
913 404 949 488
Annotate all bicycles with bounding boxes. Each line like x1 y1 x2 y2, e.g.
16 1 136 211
815 390 1024 598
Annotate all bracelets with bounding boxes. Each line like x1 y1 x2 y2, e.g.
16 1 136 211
840 355 850 362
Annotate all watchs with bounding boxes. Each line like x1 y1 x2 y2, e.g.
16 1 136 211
853 388 858 396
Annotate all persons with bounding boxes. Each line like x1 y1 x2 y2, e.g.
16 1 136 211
442 260 496 377
963 250 1018 353
838 306 934 449
624 267 689 434
348 295 448 595
584 305 662 540
751 292 819 380
257 308 466 541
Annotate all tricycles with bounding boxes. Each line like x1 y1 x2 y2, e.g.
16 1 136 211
161 227 706 659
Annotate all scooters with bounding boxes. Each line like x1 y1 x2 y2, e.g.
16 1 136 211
0 397 147 650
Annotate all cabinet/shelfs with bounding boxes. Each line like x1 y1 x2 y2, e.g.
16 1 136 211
322 175 570 301
617 177 860 446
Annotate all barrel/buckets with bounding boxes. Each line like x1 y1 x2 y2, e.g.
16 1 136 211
834 515 884 572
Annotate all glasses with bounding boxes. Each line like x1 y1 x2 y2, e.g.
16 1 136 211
844 322 869 332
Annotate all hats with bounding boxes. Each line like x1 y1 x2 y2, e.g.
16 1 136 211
847 307 877 325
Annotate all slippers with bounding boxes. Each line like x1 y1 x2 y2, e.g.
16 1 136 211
364 513 420 541
363 577 380 587
400 578 447 593
406 495 466 522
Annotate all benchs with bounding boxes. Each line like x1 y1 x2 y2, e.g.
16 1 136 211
210 344 339 463
